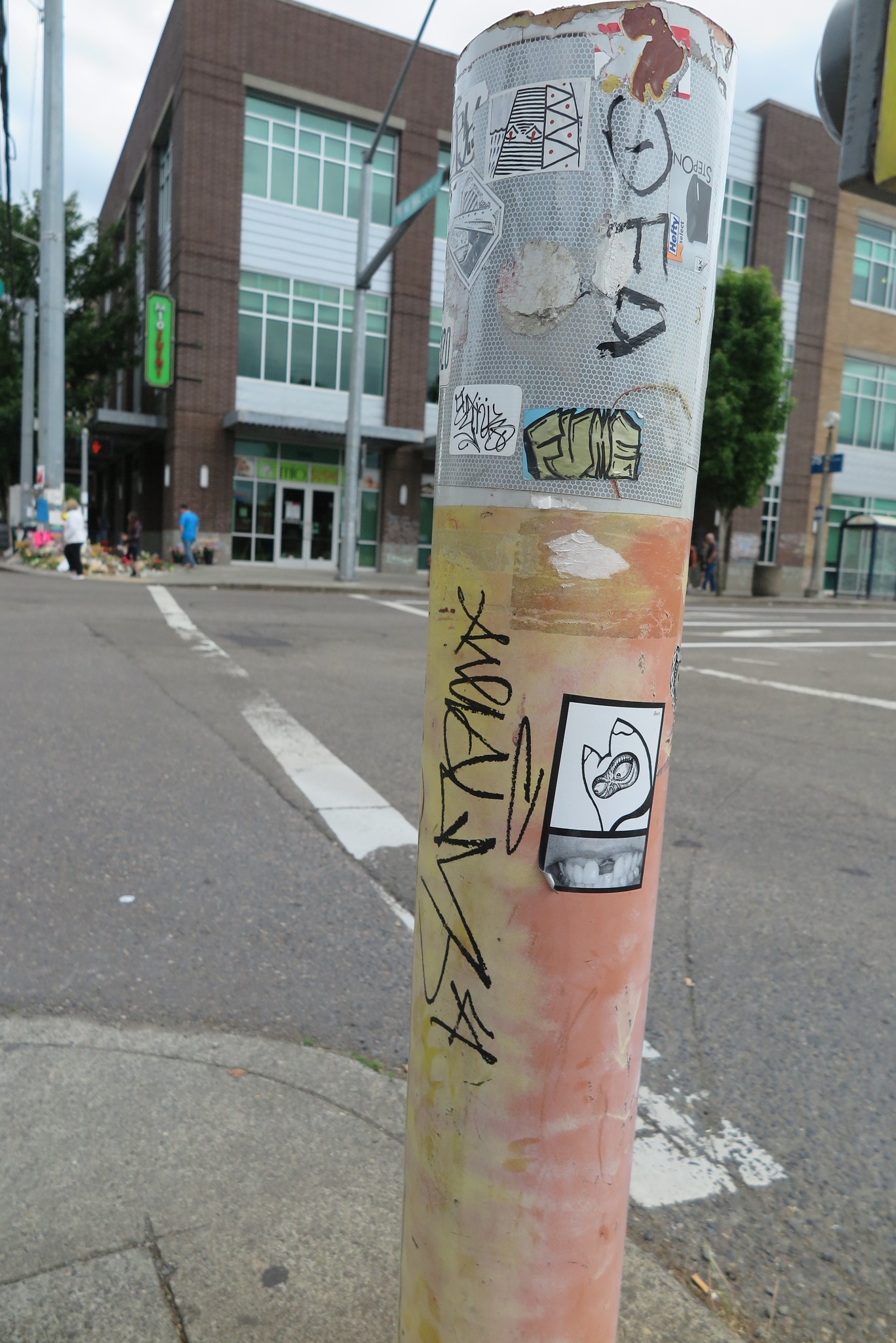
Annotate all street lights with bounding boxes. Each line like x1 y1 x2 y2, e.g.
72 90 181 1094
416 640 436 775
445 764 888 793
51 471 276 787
800 412 842 597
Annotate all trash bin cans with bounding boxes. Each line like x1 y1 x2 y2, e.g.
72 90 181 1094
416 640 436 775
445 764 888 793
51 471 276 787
10 525 37 555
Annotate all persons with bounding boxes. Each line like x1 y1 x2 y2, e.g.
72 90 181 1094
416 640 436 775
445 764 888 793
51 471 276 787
689 532 718 594
63 497 86 580
178 504 199 569
122 512 141 578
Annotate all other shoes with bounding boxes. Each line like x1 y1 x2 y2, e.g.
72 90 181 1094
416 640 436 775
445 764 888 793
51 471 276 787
72 574 85 580
130 570 137 576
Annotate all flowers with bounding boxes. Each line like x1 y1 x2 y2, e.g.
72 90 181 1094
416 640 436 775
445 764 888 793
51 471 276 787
13 532 219 577
